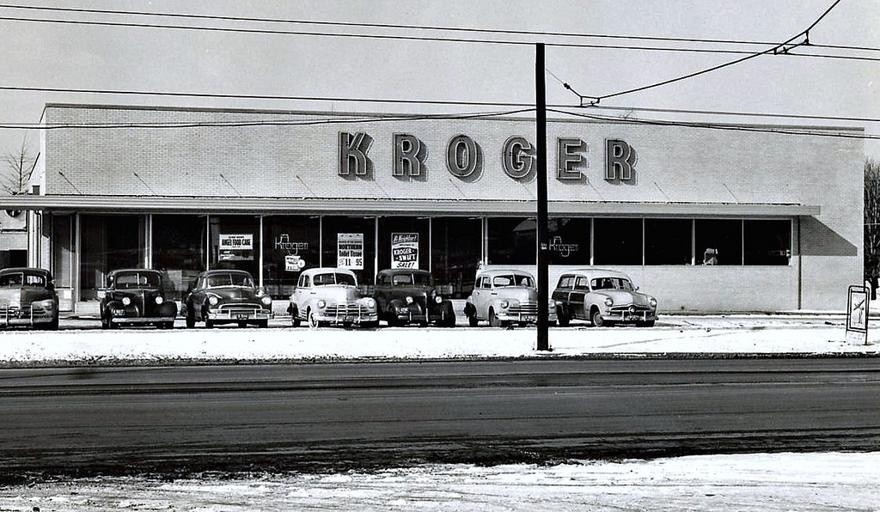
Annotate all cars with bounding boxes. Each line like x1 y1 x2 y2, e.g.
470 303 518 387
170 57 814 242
182 269 275 329
97 269 177 328
0 268 59 330
287 267 379 328
465 268 557 327
553 268 659 327
371 268 458 326
